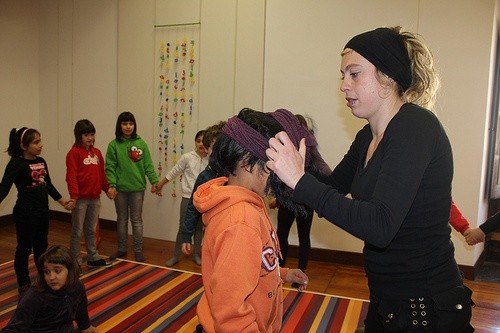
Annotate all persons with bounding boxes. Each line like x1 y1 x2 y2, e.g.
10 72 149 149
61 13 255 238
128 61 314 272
193 108 308 333
0 243 97 333
105 112 162 262
265 26 475 332
466 210 500 246
65 119 118 277
0 127 77 294
181 121 224 255
449 199 479 246
151 130 211 267
267 114 315 288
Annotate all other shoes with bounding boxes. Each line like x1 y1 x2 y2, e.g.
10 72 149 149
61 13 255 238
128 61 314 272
87 259 112 268
